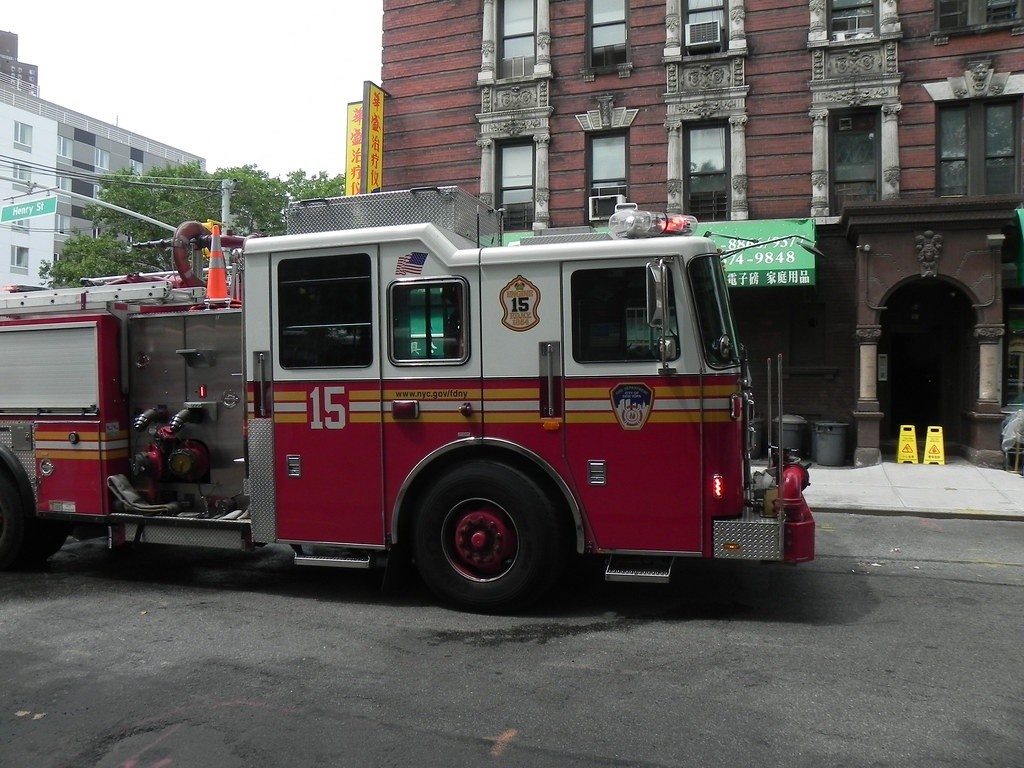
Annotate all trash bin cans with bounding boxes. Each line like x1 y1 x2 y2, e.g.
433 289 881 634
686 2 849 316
812 419 851 466
771 414 808 456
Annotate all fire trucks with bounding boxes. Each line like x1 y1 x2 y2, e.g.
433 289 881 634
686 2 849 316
0 183 819 618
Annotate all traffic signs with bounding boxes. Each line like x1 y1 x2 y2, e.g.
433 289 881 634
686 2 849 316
0 195 58 225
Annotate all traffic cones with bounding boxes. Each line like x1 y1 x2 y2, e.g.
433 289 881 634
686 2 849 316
187 224 243 312
